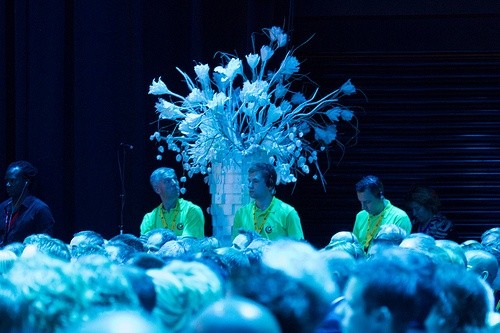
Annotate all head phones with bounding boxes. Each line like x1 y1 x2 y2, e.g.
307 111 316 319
366 177 384 198
264 164 274 187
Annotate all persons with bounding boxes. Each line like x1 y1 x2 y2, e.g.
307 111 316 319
353 175 412 254
140 167 204 239
232 163 304 242
0 161 55 249
406 186 456 242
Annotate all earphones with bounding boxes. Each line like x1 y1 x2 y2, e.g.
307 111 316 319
25 182 28 185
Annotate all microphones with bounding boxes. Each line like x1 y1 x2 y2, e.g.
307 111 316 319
121 143 133 149
